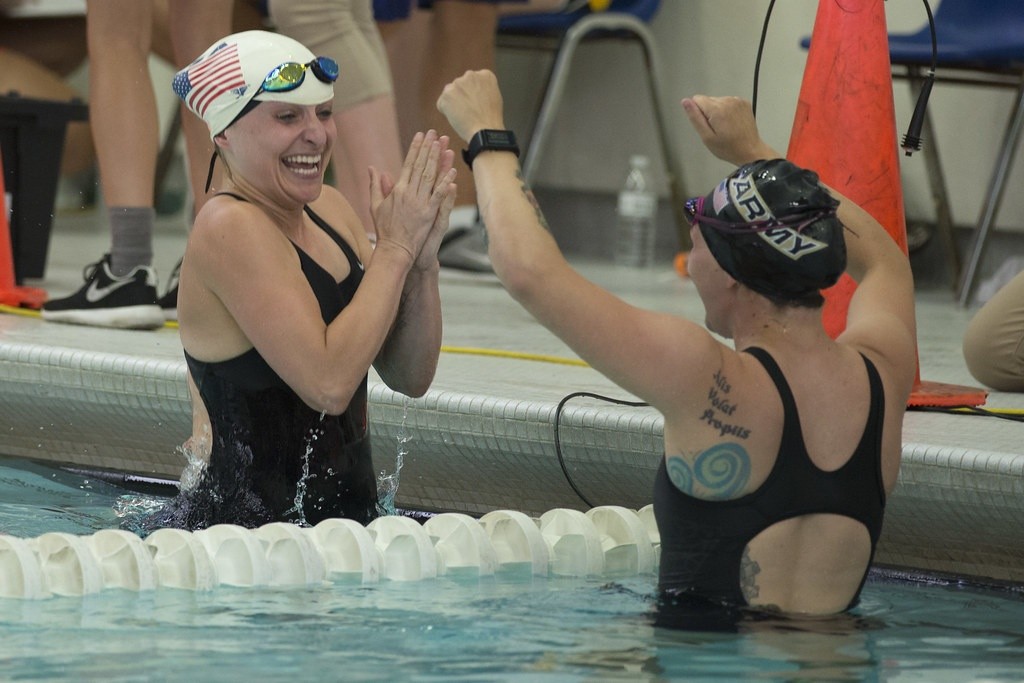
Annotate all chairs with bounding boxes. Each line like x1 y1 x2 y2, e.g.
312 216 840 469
495 0 694 251
799 0 1024 307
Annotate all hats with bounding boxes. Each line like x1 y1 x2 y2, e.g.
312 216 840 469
172 30 335 141
698 159 847 300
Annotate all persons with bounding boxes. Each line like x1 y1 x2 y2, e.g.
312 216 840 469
960 271 1024 395
172 31 458 536
40 0 404 333
437 68 917 626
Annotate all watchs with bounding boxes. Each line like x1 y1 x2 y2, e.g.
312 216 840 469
462 129 520 170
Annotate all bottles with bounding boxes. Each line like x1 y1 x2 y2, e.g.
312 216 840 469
612 155 659 270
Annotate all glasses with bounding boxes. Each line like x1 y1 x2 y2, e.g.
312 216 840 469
258 57 339 95
682 197 839 232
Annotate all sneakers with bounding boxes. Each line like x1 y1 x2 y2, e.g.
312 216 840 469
159 257 184 320
40 254 163 330
